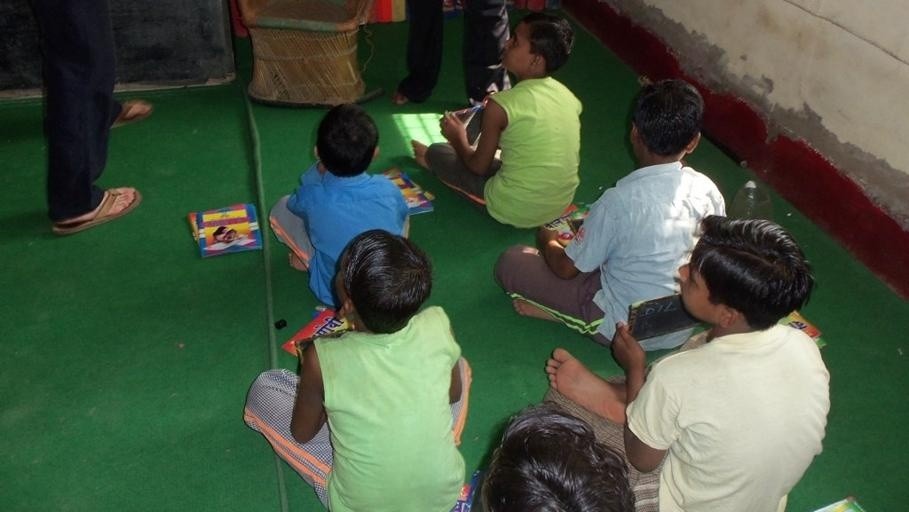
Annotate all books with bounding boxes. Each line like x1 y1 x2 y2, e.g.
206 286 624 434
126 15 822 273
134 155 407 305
454 101 482 147
776 309 828 348
383 166 437 214
281 309 355 357
629 294 712 376
547 202 591 247
186 201 262 258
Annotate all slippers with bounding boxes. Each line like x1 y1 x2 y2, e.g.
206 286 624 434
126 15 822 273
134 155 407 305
110 99 153 128
52 188 142 236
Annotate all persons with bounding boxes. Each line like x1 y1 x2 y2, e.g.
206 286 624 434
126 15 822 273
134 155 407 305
412 11 583 229
470 402 638 511
268 101 412 310
494 78 727 350
243 229 473 512
545 216 830 511
392 0 513 108
33 0 155 235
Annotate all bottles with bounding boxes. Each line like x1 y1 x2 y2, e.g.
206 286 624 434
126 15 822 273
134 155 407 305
744 180 761 217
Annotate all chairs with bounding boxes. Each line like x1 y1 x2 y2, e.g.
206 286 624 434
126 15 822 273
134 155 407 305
237 0 385 108
